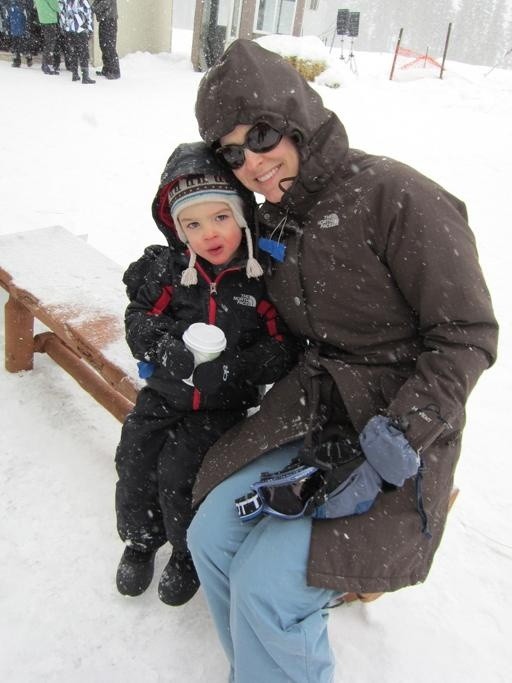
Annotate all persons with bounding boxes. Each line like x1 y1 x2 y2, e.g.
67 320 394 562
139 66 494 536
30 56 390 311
1 0 121 82
120 36 501 682
113 138 305 607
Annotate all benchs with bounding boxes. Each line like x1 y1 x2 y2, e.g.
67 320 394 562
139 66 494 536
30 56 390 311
0 223 460 606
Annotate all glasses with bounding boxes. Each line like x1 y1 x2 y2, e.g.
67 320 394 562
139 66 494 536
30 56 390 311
234 467 326 523
215 122 282 171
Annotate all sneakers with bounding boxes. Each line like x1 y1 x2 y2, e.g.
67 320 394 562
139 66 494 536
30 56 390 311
117 545 155 596
158 548 200 605
12 56 120 83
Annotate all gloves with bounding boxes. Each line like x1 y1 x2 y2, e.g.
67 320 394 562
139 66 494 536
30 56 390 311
130 314 195 378
192 349 249 396
299 415 421 519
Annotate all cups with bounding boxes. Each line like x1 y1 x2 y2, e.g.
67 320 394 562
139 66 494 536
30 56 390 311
181 323 227 389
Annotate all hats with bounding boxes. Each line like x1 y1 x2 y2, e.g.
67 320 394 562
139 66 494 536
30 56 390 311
167 174 248 244
